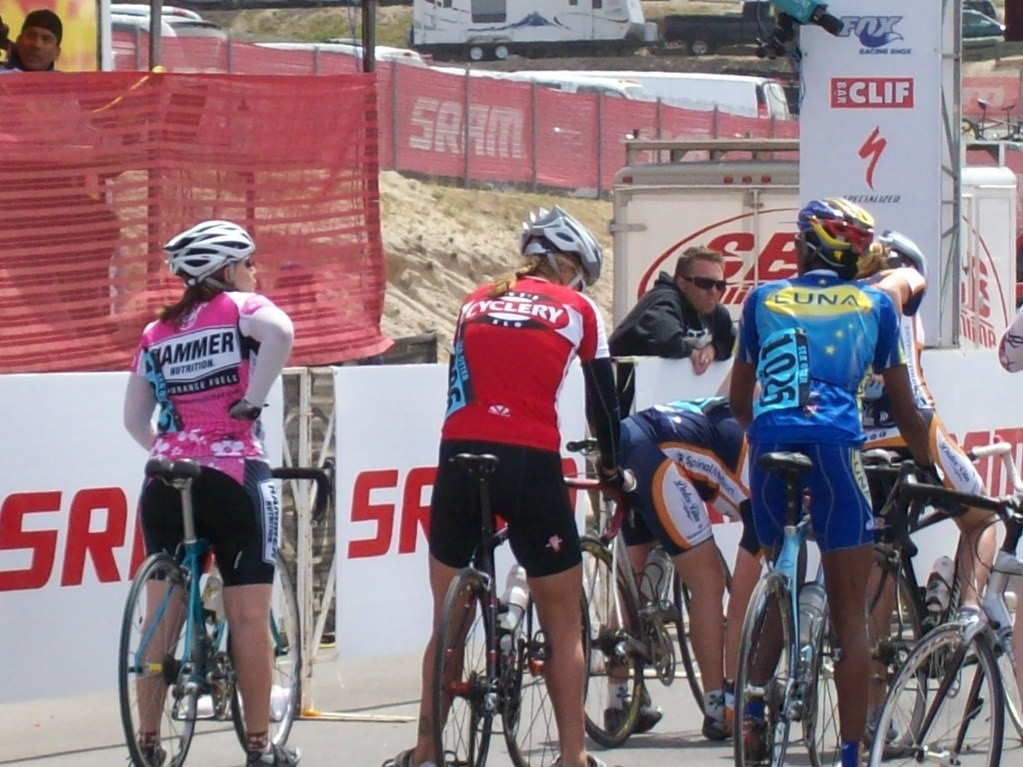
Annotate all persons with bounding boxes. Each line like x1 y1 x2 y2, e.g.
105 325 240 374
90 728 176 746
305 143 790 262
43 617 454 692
596 229 1023 741
0 2 149 333
729 195 944 767
376 204 626 767
123 221 301 767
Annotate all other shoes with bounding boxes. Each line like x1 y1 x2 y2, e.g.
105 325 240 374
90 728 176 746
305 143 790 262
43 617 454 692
247 741 300 767
129 745 167 767
740 712 771 763
382 749 439 767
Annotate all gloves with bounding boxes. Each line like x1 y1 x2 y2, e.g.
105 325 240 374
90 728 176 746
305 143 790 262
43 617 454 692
604 465 644 507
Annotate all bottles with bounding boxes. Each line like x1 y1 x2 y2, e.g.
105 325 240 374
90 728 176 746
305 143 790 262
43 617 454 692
925 554 954 614
498 561 529 644
199 553 225 633
642 546 669 601
798 579 824 641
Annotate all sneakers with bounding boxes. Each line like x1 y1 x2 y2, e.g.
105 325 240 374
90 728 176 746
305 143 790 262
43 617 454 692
605 699 664 735
702 708 736 740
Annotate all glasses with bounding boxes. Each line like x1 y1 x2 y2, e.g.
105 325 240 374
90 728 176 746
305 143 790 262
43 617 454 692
236 258 253 268
680 275 728 292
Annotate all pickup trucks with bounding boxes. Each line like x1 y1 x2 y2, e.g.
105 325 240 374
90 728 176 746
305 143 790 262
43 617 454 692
663 1 775 56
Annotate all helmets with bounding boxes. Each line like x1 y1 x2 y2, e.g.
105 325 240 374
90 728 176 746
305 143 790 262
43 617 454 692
878 229 927 313
520 204 603 285
163 219 257 286
796 197 874 268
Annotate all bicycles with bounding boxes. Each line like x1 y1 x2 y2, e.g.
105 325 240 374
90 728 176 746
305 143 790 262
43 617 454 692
568 438 733 747
431 435 635 766
735 434 1023 766
118 457 335 767
